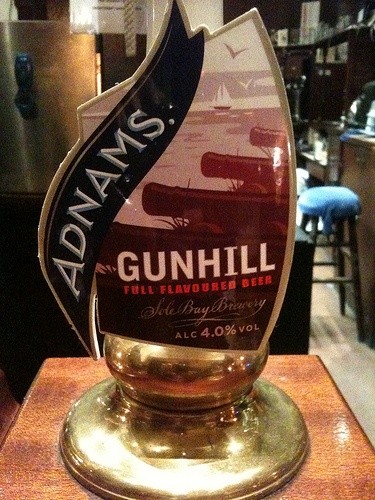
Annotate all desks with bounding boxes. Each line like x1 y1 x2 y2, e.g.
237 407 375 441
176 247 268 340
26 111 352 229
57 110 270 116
1 353 375 499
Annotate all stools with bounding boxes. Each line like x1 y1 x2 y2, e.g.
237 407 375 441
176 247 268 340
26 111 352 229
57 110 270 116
297 185 365 342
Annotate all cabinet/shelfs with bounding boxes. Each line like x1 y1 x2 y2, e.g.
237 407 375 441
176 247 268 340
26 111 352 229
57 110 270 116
275 24 375 126
1 20 103 200
334 134 375 347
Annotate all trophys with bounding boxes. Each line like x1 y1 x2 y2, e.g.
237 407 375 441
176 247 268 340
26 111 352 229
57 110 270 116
37 0 312 500
